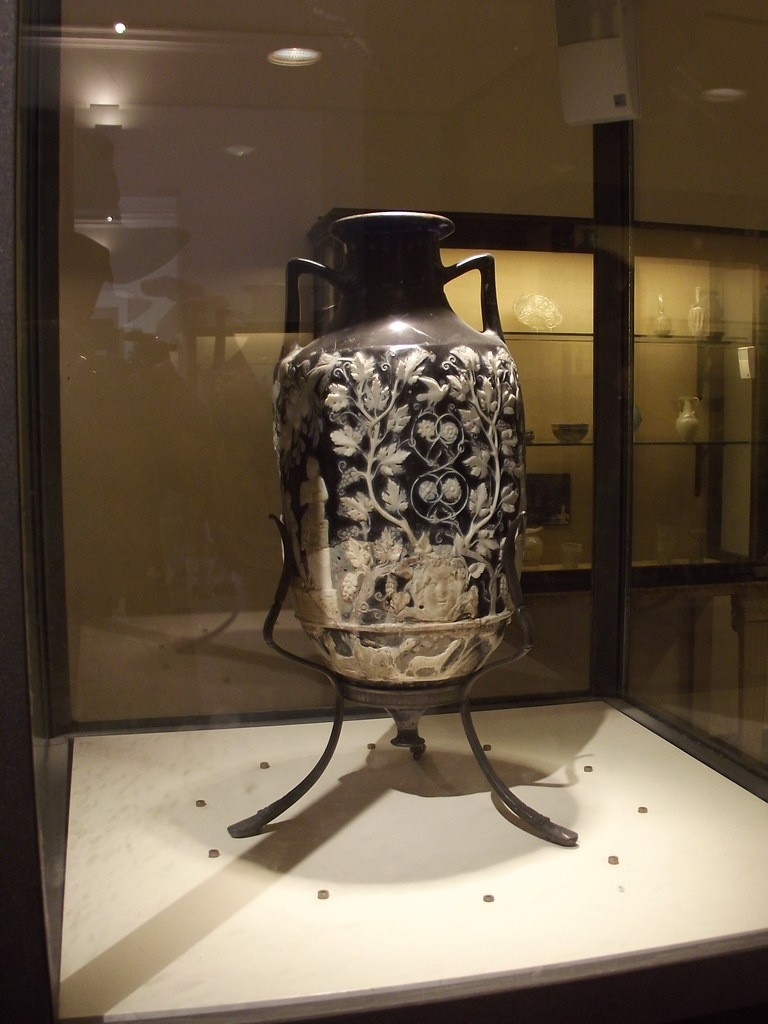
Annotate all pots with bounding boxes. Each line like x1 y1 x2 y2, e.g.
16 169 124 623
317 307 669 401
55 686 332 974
551 423 589 441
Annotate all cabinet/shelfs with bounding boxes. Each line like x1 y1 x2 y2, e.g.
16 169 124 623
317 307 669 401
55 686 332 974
622 218 767 588
306 207 620 588
